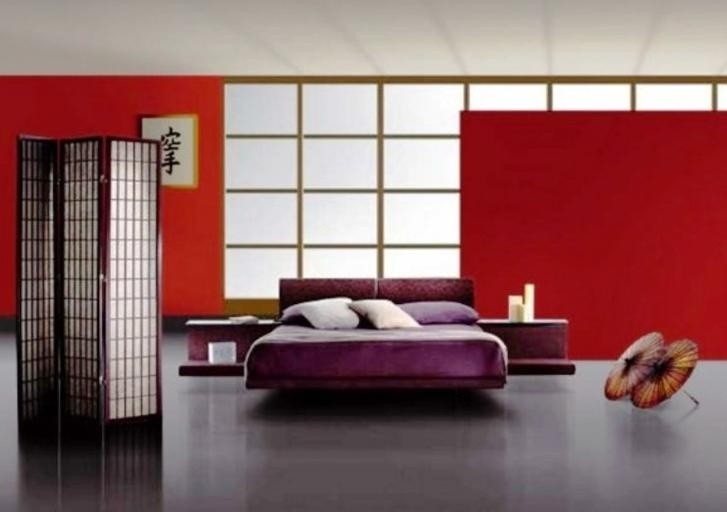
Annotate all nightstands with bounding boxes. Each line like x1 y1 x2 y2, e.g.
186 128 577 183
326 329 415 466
476 318 575 375
178 318 282 377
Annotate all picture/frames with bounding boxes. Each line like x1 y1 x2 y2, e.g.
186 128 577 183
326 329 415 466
138 113 198 192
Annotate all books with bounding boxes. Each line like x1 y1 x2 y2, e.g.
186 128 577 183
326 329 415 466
229 313 258 324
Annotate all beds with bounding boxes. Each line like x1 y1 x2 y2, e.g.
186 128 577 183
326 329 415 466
244 278 508 391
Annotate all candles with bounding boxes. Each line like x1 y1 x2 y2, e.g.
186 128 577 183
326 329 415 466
507 280 535 323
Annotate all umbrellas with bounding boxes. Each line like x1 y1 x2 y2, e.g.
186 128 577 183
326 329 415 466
603 331 662 400
629 339 702 407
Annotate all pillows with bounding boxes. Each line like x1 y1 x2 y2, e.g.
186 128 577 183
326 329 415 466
279 296 479 331
